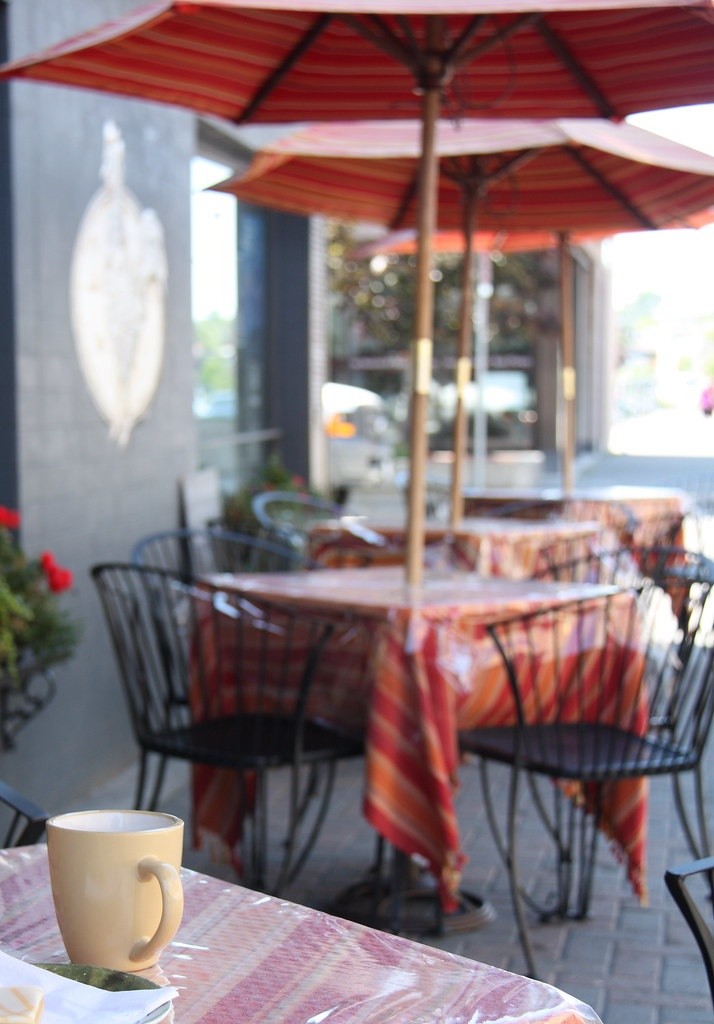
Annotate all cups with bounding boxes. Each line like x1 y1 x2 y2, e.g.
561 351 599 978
45 810 184 972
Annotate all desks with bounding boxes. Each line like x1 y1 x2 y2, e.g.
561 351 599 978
460 480 685 567
299 511 611 590
0 842 601 1023
195 563 644 932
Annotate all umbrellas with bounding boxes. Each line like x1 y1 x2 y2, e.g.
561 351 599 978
1 1 712 579
345 204 713 451
199 118 714 519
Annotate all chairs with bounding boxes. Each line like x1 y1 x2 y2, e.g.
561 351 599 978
253 487 358 576
543 507 688 618
399 467 458 527
488 497 640 537
479 548 713 923
206 520 344 848
87 563 392 917
457 577 713 985
128 528 388 890
